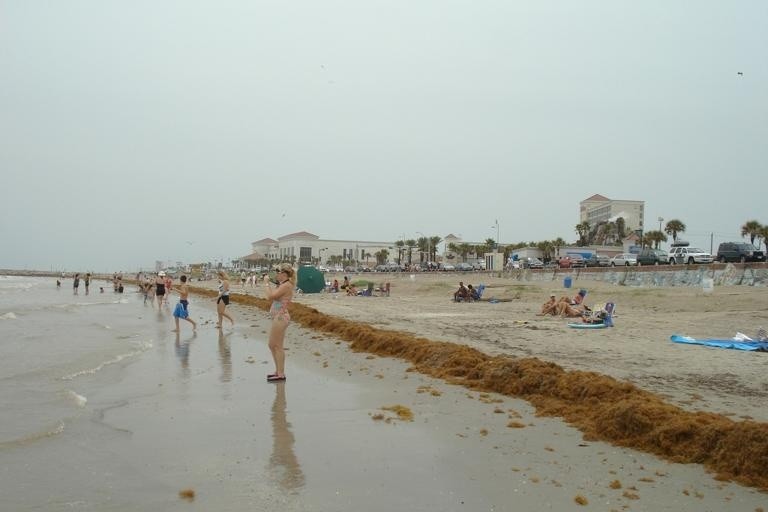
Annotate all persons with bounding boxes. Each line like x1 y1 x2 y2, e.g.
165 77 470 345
537 291 613 324
454 282 469 303
170 274 196 332
384 263 441 272
330 275 358 296
54 269 270 307
214 271 234 328
263 263 295 382
466 285 478 303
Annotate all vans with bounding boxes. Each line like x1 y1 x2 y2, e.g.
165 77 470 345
636 249 668 266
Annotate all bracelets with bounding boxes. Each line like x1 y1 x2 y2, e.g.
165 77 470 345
265 283 269 287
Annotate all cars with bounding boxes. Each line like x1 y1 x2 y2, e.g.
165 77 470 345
506 261 519 269
304 261 486 272
559 254 585 268
585 254 613 268
611 253 636 267
519 256 543 269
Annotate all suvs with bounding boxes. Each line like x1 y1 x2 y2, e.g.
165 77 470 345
667 241 714 264
716 241 764 263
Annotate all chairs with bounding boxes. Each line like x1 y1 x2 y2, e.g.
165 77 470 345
357 282 391 297
561 288 588 309
468 283 486 301
585 302 617 325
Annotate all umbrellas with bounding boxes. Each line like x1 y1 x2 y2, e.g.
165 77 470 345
296 267 326 293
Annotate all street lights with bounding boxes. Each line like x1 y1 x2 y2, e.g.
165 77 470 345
319 248 325 271
657 216 665 250
325 247 328 267
